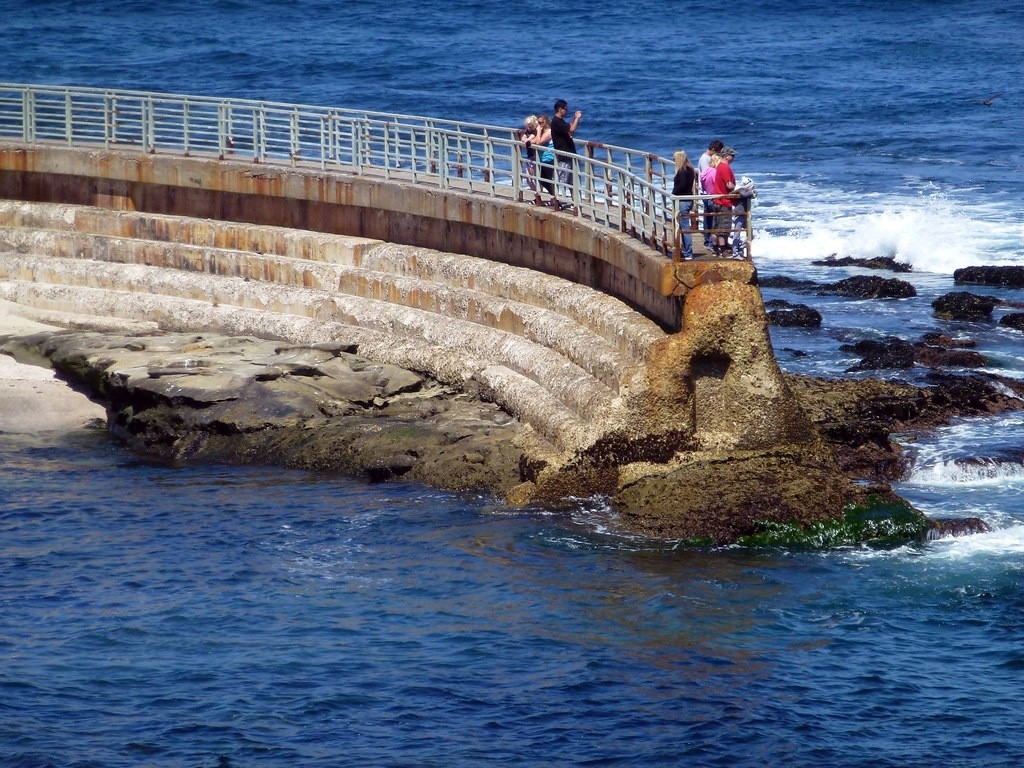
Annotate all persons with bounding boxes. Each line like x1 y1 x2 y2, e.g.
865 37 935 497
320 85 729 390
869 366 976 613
521 114 556 205
670 139 758 260
551 99 582 209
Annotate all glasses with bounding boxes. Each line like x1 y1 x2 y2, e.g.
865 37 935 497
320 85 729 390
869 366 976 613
561 108 568 111
538 121 544 123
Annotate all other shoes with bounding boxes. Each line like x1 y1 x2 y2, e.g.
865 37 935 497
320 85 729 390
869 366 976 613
529 199 536 205
733 254 747 261
680 254 693 260
719 251 733 259
712 250 723 257
704 245 713 251
571 203 574 209
545 198 555 206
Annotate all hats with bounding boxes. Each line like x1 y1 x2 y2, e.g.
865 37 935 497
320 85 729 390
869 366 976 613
721 146 737 155
